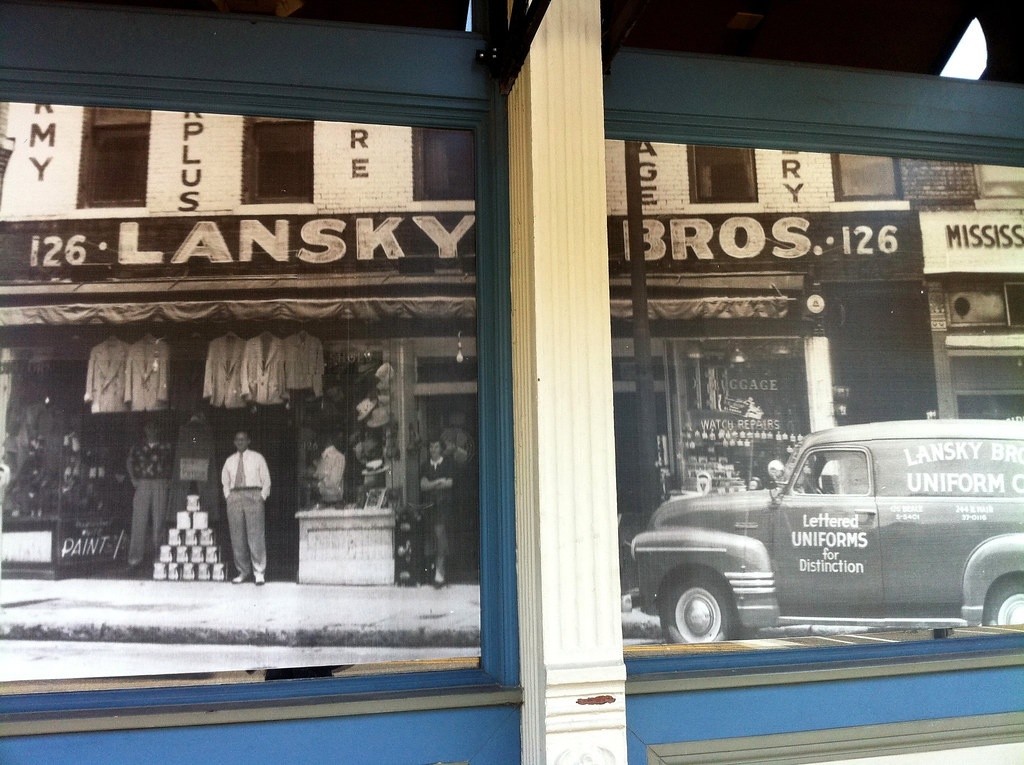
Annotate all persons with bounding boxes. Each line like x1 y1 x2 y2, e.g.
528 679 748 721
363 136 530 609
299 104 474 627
220 431 272 585
312 445 345 503
126 420 172 567
419 437 453 585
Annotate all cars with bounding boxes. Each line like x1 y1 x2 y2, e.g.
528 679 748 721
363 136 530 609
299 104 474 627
629 420 1024 647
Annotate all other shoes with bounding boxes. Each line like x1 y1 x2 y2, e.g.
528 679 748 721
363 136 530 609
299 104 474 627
232 576 246 583
255 576 264 585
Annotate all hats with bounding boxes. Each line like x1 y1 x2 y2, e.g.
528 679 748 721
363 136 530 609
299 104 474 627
356 362 395 428
362 460 390 475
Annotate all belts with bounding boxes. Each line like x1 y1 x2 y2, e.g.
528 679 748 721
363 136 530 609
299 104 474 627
230 487 262 491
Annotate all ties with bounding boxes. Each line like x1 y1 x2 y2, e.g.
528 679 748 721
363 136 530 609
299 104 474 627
234 453 245 488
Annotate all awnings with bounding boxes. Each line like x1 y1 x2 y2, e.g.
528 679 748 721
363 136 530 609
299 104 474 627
0 298 793 326
